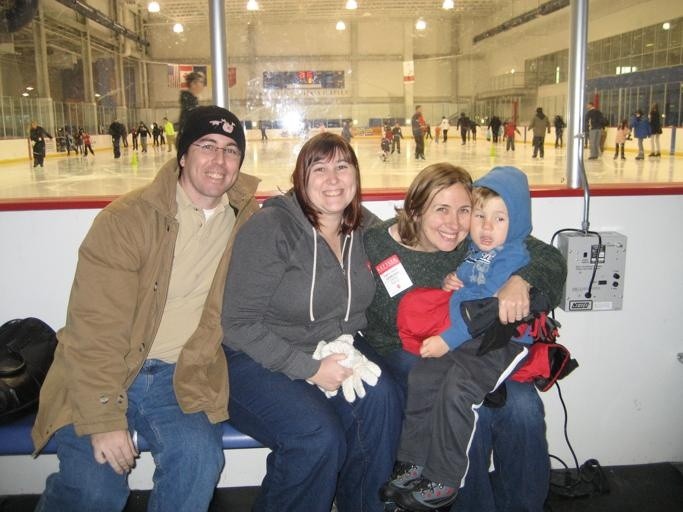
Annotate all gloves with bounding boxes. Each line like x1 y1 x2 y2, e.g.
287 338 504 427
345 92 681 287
307 334 382 403
461 288 552 357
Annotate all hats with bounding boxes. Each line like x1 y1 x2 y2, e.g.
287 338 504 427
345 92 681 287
177 104 246 164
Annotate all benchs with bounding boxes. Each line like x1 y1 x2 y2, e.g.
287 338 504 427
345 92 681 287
0 412 316 512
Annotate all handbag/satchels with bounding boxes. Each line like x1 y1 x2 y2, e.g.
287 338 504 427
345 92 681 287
1 317 63 429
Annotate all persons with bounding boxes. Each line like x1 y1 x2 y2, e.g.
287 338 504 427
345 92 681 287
380 119 404 162
417 113 432 139
163 117 177 152
379 165 532 511
583 102 609 159
180 72 207 133
340 119 353 142
259 124 268 140
631 110 651 160
109 118 121 158
28 105 262 512
456 111 479 145
151 122 159 147
487 115 522 151
121 124 128 147
72 126 83 155
363 162 567 511
159 125 165 145
434 125 441 144
648 103 663 156
441 116 449 143
130 127 138 150
216 132 407 512
554 115 567 149
30 121 52 168
613 120 630 159
82 133 95 156
528 107 551 158
411 105 427 160
64 125 78 155
135 121 151 153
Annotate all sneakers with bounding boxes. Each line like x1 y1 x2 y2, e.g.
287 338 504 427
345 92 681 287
394 479 460 512
379 461 425 504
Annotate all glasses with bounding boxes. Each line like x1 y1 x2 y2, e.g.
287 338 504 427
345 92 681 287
192 143 242 158
